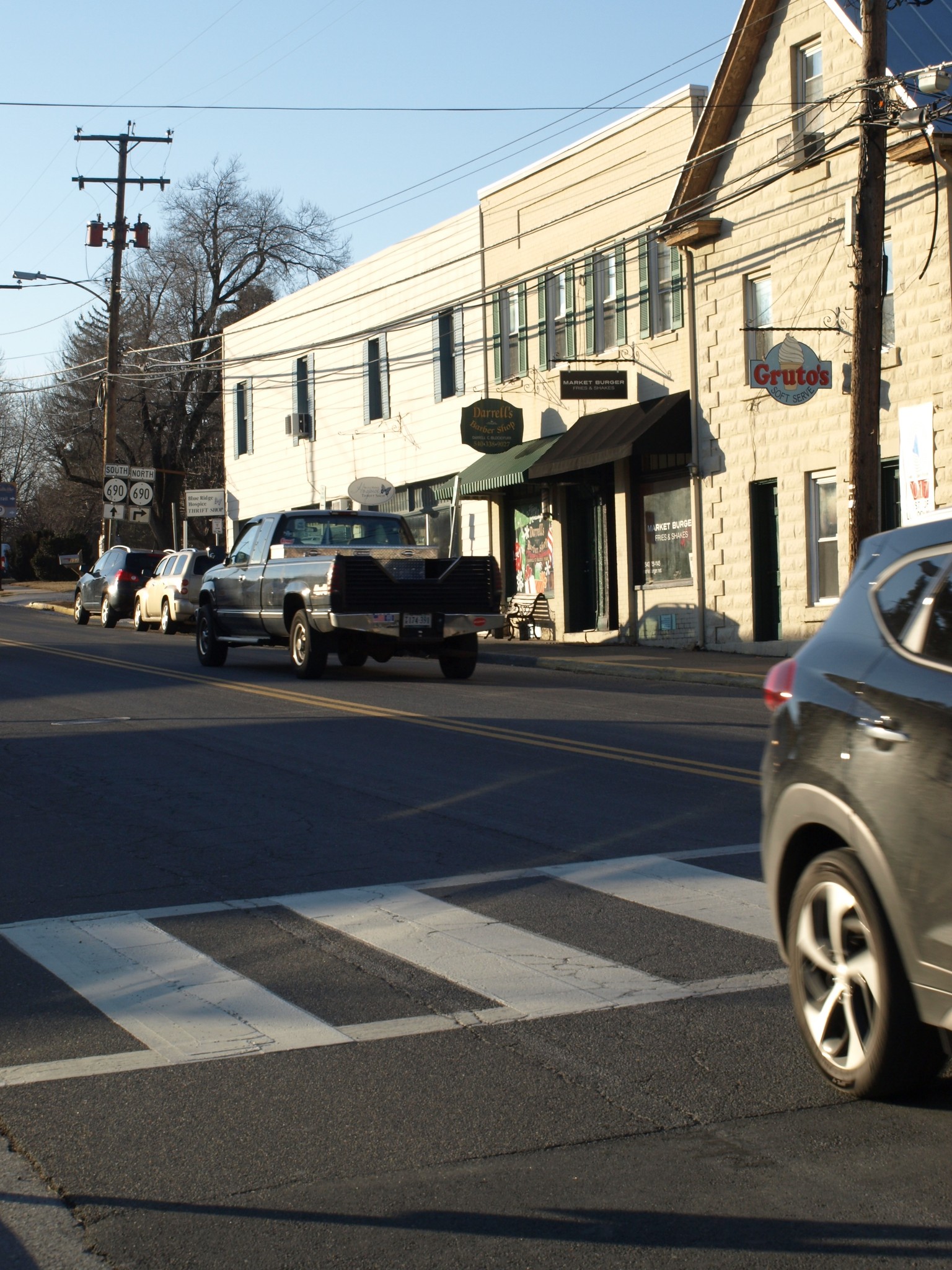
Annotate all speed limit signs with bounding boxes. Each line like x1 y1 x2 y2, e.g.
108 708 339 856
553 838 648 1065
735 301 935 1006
128 480 155 507
102 476 129 505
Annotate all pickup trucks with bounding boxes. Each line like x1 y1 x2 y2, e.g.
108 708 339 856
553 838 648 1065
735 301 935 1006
194 510 505 681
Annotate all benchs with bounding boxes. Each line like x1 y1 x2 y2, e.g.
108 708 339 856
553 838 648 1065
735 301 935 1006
484 593 542 640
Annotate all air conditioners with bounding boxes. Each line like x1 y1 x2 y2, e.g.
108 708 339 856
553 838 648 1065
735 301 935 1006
776 131 824 168
285 413 309 436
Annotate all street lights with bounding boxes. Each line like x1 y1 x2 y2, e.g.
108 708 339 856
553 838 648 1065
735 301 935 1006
13 120 173 556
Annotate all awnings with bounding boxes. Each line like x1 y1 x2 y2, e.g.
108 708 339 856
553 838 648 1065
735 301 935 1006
528 389 693 479
434 431 563 502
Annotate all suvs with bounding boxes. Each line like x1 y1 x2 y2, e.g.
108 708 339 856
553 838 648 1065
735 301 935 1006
758 518 952 1101
74 545 177 629
133 548 232 635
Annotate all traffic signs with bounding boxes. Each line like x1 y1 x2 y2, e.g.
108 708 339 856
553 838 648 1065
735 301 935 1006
104 463 130 477
212 518 222 534
0 482 16 518
130 467 155 482
102 503 126 521
127 507 150 524
